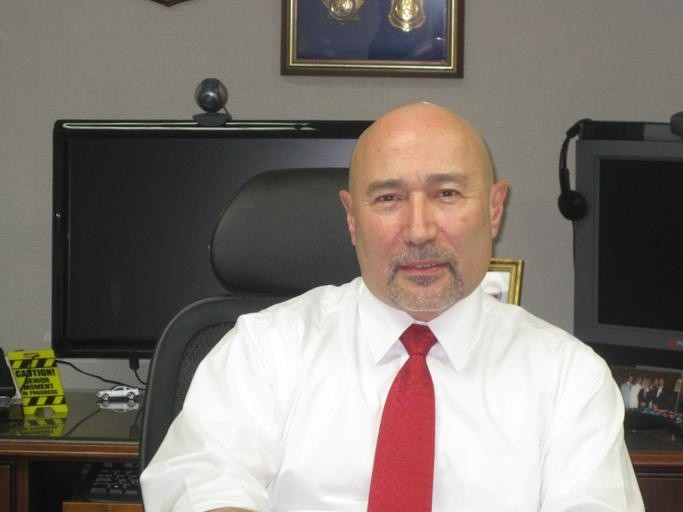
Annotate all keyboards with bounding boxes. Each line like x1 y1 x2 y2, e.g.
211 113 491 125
82 460 142 502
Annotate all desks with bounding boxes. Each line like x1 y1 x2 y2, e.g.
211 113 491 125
0 391 683 512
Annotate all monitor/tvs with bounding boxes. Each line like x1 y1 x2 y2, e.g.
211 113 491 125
572 121 683 369
50 119 375 360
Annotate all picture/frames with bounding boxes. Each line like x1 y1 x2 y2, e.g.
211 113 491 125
477 257 524 306
613 366 683 442
282 0 464 78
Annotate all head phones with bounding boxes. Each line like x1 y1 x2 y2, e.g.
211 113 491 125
557 120 592 219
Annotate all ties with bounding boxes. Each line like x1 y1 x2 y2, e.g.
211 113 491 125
364 323 439 511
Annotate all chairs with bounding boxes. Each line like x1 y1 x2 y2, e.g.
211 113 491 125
140 168 361 476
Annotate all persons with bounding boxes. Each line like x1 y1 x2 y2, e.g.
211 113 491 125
137 100 648 512
617 375 667 432
